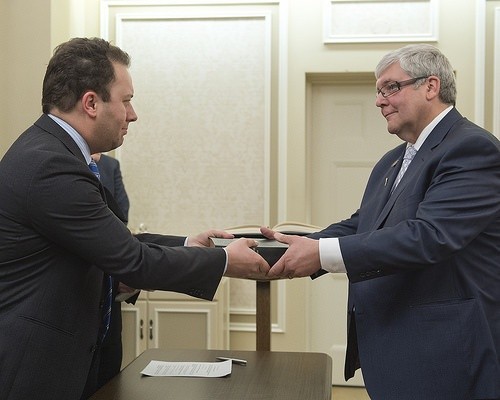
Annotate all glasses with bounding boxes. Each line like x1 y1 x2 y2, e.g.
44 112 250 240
376 75 432 98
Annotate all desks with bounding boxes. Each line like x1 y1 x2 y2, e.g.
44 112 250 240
87 347 332 399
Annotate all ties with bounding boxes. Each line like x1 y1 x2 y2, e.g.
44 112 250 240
390 146 417 196
90 159 112 341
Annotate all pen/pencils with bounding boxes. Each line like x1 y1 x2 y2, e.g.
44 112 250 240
216 357 247 366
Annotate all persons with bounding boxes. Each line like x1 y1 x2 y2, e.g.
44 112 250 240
0 35 270 400
89 151 141 383
260 44 500 400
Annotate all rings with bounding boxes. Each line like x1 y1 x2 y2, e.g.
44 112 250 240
288 275 294 279
